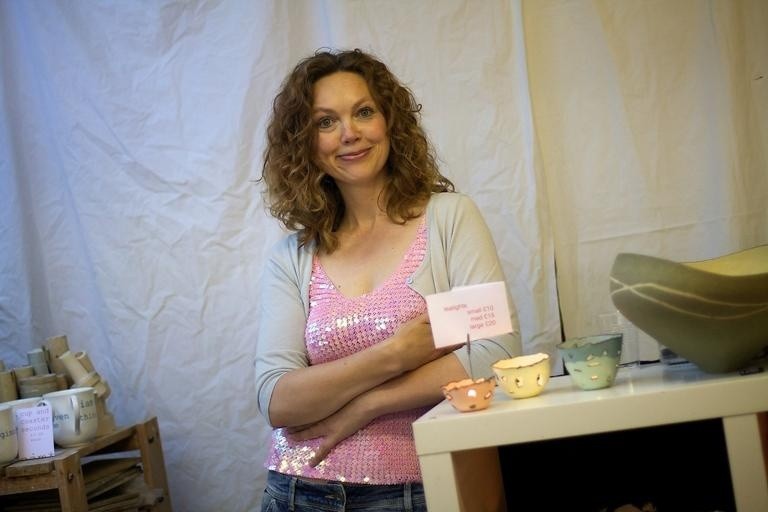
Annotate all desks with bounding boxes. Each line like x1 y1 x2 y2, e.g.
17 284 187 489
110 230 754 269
411 357 768 511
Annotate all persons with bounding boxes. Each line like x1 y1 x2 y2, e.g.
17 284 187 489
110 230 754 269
252 49 519 511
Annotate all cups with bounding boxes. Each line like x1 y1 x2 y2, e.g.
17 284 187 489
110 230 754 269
0 397 51 467
598 310 641 373
40 386 97 446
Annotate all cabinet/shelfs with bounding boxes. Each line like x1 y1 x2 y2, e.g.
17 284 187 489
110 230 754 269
0 415 174 512
